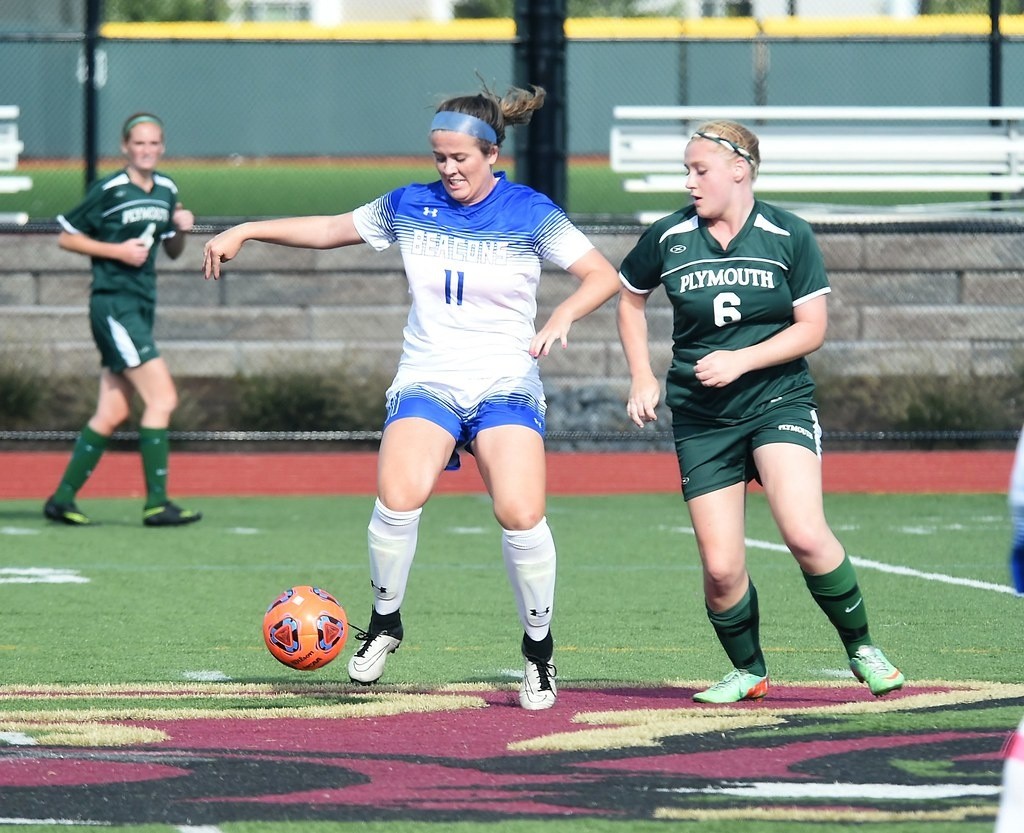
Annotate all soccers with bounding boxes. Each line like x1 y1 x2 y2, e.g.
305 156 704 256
262 584 349 672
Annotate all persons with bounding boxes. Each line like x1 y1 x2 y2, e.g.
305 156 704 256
616 121 906 706
202 68 621 712
43 112 199 527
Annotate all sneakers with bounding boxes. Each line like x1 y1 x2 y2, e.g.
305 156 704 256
44 495 96 525
518 639 557 711
142 502 202 526
849 644 904 698
693 667 769 703
347 620 403 687
